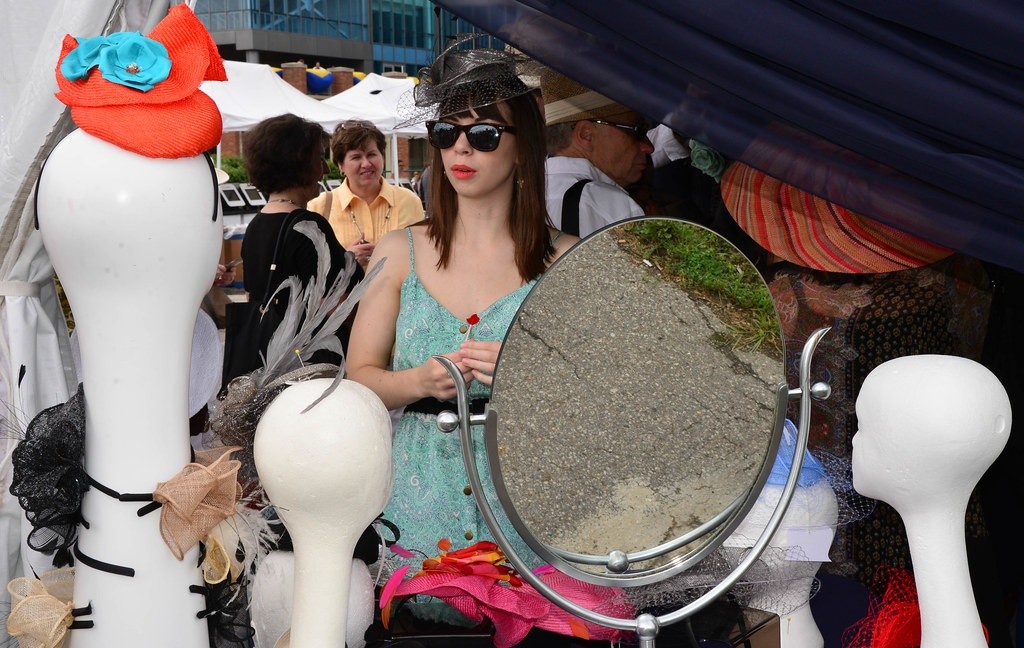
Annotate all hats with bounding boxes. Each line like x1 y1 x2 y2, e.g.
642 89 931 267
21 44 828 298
513 59 641 126
393 31 553 129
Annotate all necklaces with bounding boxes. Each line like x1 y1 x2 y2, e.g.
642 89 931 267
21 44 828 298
267 198 300 206
347 201 392 263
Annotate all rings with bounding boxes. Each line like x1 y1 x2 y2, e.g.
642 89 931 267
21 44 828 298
368 256 371 261
218 276 222 281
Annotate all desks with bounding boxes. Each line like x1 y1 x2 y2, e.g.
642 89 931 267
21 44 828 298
722 419 836 648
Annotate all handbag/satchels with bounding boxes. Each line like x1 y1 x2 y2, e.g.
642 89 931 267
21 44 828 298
217 209 311 399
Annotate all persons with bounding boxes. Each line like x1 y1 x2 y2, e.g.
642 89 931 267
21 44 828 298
344 34 581 581
214 113 431 400
721 117 993 594
540 64 709 241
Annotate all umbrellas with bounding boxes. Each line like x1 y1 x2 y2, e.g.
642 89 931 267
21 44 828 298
196 58 442 189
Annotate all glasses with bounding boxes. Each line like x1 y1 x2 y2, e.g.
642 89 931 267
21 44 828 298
342 121 376 130
425 119 517 152
590 119 649 140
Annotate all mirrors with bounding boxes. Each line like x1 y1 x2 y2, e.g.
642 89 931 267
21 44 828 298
433 216 831 647
